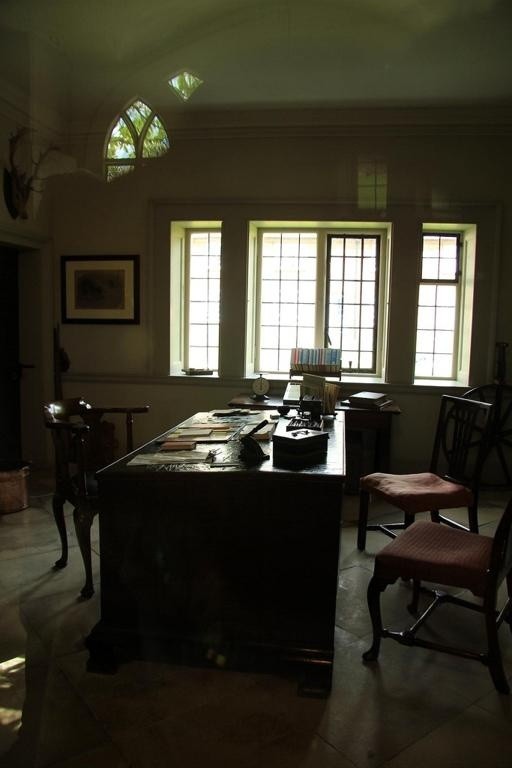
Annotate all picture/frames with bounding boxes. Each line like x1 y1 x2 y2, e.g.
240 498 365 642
57 251 142 326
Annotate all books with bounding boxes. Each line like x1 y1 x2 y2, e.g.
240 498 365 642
153 373 394 451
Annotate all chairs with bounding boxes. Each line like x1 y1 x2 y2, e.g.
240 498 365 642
358 394 495 553
39 394 150 604
361 498 510 699
354 391 511 699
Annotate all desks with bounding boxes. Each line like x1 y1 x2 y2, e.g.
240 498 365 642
80 409 348 692
229 394 401 499
